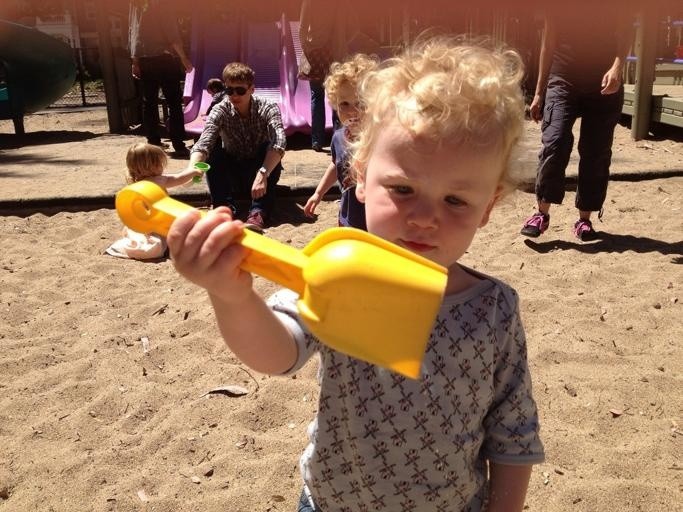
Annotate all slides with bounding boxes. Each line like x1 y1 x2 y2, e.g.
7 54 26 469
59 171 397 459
165 11 334 137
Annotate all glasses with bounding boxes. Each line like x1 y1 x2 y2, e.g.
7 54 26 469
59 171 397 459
222 84 251 97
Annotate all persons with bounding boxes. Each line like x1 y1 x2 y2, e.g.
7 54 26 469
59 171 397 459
106 144 204 261
128 0 194 158
165 31 546 512
205 79 226 114
298 1 351 152
520 0 636 237
302 54 379 230
187 62 287 228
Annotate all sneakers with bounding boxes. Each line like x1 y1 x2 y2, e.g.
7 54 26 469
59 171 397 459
520 212 550 238
310 140 323 153
239 206 267 235
573 218 598 242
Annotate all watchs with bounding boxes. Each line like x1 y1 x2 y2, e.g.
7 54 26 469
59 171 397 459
258 167 268 176
